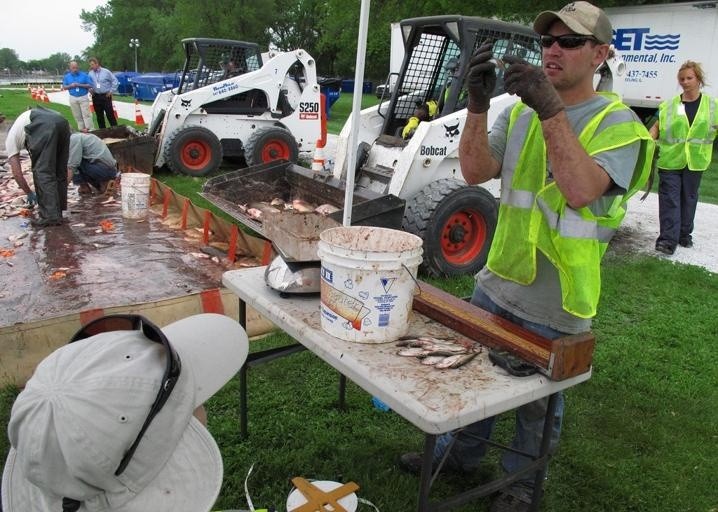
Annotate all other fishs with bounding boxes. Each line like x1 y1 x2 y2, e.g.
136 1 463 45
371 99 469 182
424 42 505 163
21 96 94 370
395 335 482 370
238 197 342 223
136 203 262 268
0 148 122 283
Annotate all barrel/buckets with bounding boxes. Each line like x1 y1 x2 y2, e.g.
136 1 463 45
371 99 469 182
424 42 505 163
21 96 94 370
315 225 425 346
119 171 152 220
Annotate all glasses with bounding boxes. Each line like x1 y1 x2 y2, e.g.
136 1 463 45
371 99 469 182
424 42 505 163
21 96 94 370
68 313 182 476
539 32 597 50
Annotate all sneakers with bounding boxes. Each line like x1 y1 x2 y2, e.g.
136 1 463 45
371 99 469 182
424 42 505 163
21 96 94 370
491 480 544 511
656 240 673 254
99 180 116 198
78 185 91 194
32 217 63 228
401 437 487 483
680 235 693 247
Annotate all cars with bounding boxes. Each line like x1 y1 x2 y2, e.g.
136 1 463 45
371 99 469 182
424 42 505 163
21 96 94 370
374 85 396 99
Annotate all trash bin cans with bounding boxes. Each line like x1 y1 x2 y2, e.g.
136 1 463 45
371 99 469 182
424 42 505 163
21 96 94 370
364 81 372 94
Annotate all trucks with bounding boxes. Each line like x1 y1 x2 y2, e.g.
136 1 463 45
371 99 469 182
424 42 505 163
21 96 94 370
486 0 718 119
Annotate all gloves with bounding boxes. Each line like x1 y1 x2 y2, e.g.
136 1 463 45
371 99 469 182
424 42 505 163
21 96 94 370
467 38 496 113
503 55 566 122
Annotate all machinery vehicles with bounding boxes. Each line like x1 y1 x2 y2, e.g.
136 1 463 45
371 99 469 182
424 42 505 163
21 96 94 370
190 12 629 280
86 35 330 184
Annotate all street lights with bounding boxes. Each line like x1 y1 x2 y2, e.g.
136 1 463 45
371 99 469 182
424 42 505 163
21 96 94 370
129 38 140 73
3 67 11 76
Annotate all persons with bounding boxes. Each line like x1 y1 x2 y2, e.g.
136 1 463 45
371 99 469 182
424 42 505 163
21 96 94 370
62 60 94 131
649 59 717 255
398 1 654 510
5 105 70 228
401 55 468 137
88 56 118 127
66 132 119 192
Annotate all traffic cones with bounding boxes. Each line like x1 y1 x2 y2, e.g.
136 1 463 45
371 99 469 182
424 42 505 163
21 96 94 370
87 92 95 113
134 98 149 126
112 102 118 119
310 138 326 172
27 82 64 103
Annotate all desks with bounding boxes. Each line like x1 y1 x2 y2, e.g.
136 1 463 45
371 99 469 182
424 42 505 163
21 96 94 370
222 261 598 511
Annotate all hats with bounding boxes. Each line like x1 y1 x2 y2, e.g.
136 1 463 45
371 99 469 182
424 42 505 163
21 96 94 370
533 0 612 46
